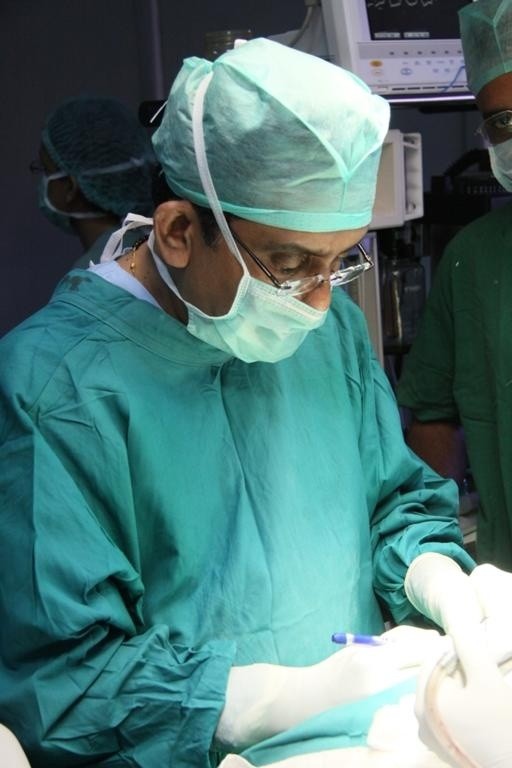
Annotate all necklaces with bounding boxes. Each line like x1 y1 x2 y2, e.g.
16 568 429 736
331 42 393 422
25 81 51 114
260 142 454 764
125 233 147 278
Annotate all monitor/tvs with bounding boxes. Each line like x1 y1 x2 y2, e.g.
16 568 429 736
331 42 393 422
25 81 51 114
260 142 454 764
282 0 475 100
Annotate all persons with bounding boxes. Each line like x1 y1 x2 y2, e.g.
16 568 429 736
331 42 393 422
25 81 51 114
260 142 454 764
1 34 510 767
398 1 511 570
29 95 155 272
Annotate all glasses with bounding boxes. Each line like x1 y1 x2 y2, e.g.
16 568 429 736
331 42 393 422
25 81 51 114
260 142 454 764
473 108 512 145
229 226 378 298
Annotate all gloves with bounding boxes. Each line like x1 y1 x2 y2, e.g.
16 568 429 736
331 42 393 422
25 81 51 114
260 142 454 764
401 548 490 635
216 622 445 754
417 634 511 768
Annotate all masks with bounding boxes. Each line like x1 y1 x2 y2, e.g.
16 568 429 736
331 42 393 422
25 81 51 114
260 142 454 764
483 137 512 194
185 269 330 365
